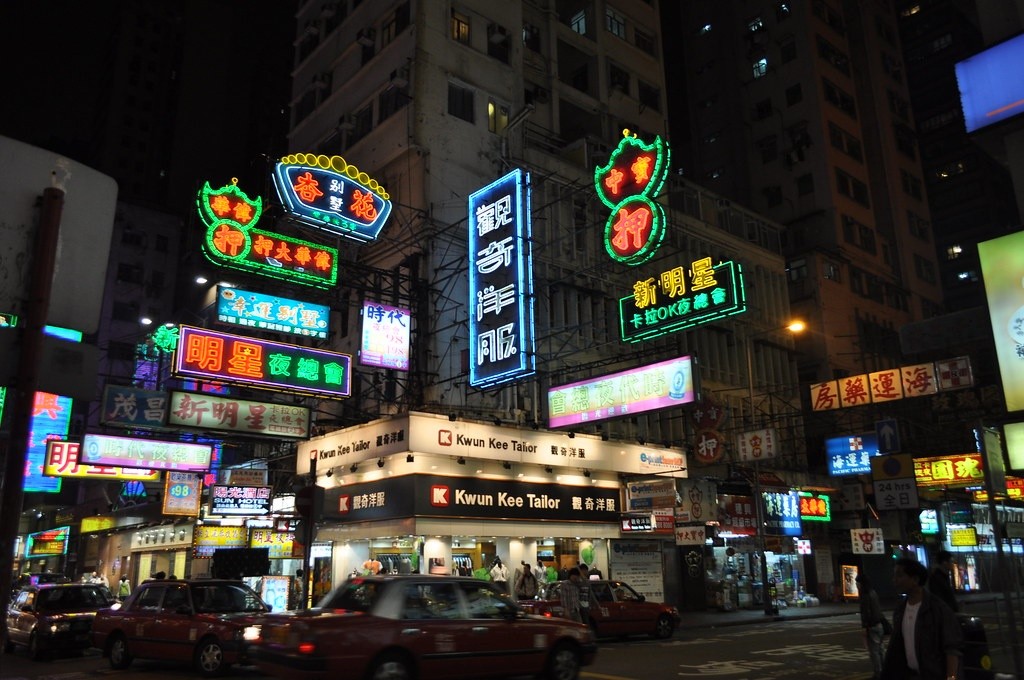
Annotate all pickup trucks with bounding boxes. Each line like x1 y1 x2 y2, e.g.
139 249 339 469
514 580 681 642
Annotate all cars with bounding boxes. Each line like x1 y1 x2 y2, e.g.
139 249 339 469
89 578 275 677
1 583 113 662
254 572 598 680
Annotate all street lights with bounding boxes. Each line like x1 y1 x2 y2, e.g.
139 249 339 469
741 320 805 618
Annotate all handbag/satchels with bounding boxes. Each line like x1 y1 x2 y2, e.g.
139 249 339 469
881 618 893 635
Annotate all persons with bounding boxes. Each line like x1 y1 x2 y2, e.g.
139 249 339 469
348 557 383 579
560 567 582 623
459 561 472 576
142 571 193 581
928 550 960 617
490 560 510 593
880 558 962 680
856 571 886 679
586 565 603 580
293 569 305 593
514 562 538 601
533 561 548 582
514 560 526 583
117 572 132 602
578 563 589 579
78 571 110 589
398 556 412 574
557 564 569 580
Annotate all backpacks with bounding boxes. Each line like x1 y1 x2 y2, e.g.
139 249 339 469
119 584 129 597
